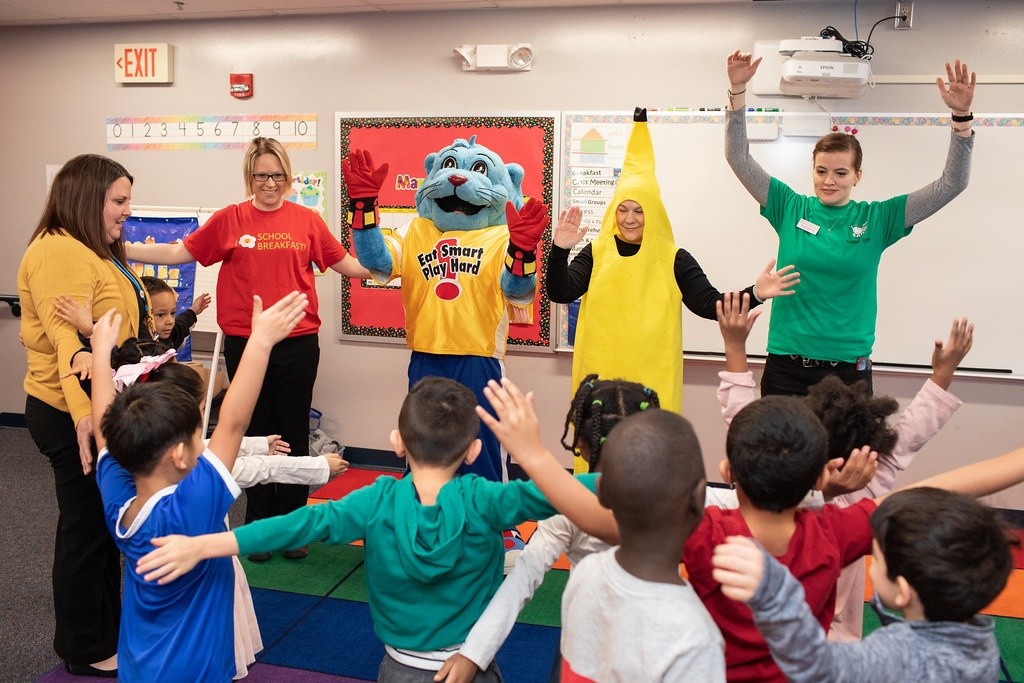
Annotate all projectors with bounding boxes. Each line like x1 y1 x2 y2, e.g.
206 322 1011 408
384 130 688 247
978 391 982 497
780 60 870 97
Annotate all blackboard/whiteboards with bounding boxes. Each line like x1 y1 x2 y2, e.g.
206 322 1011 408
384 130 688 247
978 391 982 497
552 107 1024 384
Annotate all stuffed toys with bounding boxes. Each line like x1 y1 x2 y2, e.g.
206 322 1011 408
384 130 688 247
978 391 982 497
343 138 551 577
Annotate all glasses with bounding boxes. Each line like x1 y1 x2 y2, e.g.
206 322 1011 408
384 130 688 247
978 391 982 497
252 172 287 181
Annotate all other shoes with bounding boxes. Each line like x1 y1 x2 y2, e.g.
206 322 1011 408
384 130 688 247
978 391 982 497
65 661 118 677
247 549 273 561
285 545 308 558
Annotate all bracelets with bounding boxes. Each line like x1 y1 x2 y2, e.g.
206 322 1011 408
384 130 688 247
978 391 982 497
951 111 973 122
728 89 746 113
951 125 972 132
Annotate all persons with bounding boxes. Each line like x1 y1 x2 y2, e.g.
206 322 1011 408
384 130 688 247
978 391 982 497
119 136 373 560
50 276 349 683
432 291 1024 683
544 199 801 473
134 376 604 683
723 48 977 394
17 153 158 677
